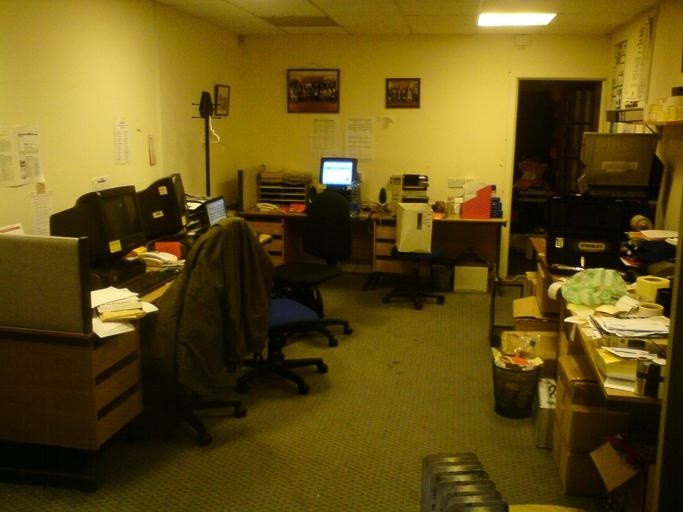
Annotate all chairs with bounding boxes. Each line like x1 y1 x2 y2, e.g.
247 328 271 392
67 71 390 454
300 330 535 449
123 219 274 448
271 190 354 342
225 219 328 396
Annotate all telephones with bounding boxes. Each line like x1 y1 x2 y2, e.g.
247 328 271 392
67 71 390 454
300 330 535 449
255 202 285 213
139 250 178 267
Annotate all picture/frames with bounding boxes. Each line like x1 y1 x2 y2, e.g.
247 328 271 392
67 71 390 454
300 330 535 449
216 84 233 116
386 77 422 109
286 67 341 114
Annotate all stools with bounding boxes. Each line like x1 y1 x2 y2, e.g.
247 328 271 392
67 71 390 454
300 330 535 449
383 246 443 310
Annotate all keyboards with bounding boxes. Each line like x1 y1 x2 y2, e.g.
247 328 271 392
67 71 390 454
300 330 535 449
116 269 181 297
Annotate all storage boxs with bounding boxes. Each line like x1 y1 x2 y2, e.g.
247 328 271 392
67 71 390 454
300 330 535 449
523 272 536 295
532 378 556 448
501 331 558 377
534 264 562 314
553 382 630 498
530 280 537 294
454 264 488 294
513 296 560 335
589 438 650 512
559 355 624 405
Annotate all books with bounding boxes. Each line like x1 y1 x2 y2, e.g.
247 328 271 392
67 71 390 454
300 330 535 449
594 345 668 393
90 286 159 338
563 314 672 351
261 170 283 183
491 184 503 219
594 295 639 318
284 170 310 185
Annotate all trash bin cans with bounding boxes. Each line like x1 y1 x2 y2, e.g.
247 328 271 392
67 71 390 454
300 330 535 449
492 351 545 418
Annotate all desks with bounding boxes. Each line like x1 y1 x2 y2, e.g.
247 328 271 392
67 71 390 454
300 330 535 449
0 231 275 451
523 237 661 401
243 205 506 289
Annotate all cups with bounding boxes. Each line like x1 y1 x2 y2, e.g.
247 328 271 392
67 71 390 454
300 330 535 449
640 303 663 318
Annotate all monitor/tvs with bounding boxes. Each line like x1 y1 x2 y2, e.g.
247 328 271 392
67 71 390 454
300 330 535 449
50 183 148 269
319 156 356 191
192 196 229 232
134 172 189 237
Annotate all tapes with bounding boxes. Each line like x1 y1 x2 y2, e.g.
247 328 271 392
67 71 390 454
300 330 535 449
638 303 664 316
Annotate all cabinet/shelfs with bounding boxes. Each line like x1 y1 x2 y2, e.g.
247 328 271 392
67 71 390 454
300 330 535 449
625 118 683 125
